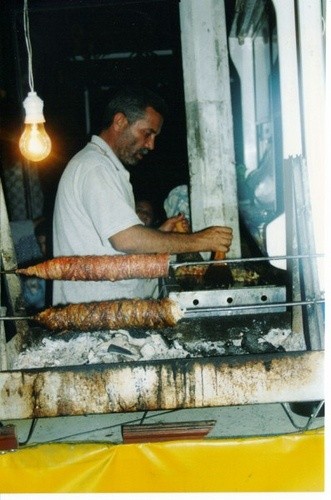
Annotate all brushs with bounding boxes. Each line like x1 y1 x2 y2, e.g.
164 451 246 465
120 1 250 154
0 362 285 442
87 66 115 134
203 231 235 289
175 222 204 263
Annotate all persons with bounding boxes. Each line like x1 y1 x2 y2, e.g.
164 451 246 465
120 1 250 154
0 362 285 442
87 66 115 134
133 198 155 225
32 220 53 258
52 90 233 306
